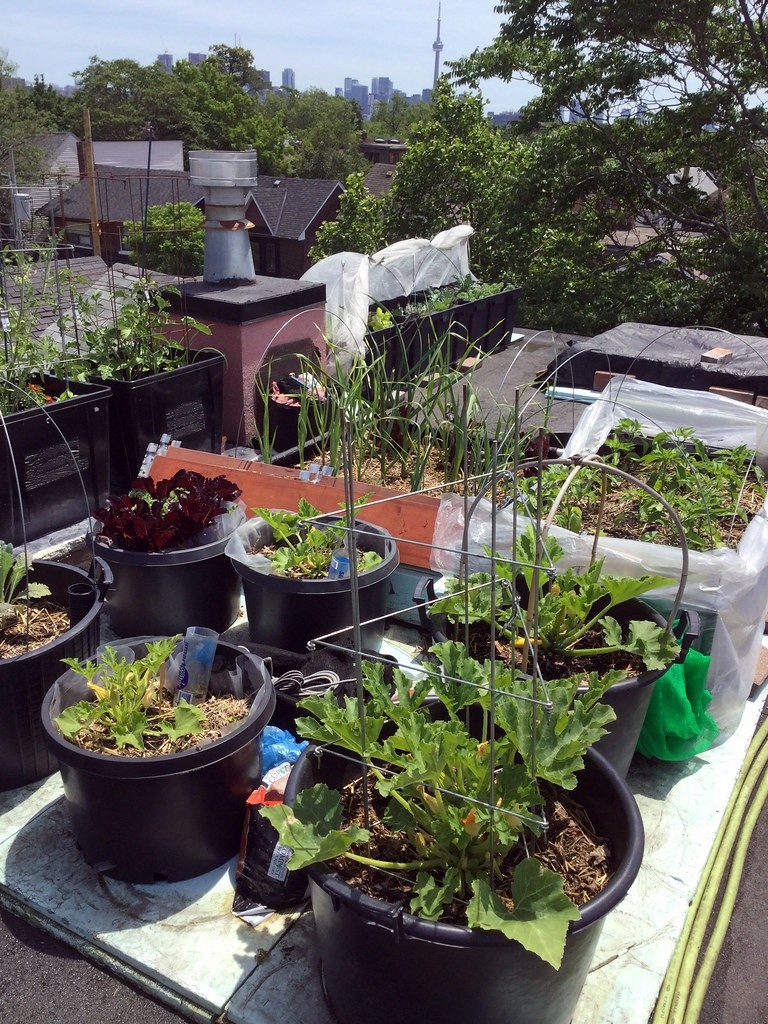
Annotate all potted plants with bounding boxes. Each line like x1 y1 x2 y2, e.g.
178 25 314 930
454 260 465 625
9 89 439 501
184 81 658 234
0 242 768 1024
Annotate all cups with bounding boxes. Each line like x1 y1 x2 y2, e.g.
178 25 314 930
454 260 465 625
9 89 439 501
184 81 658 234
327 547 360 580
173 626 220 707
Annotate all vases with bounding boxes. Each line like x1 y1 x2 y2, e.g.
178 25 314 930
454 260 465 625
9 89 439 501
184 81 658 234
269 385 341 469
